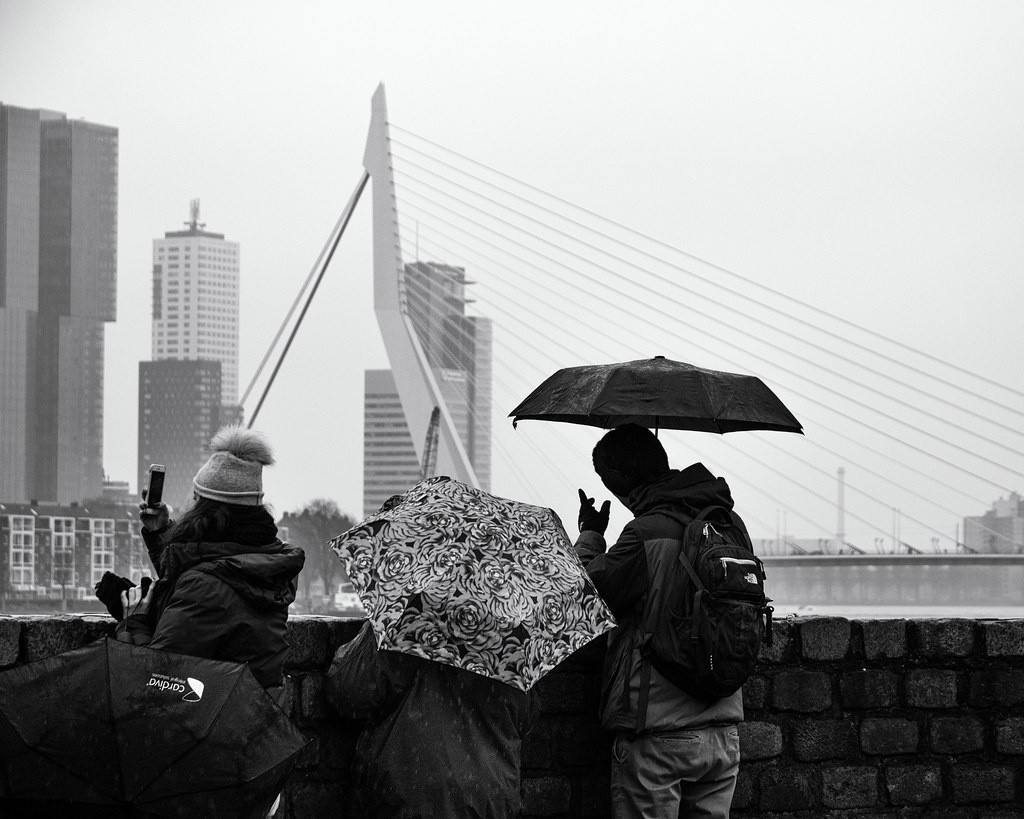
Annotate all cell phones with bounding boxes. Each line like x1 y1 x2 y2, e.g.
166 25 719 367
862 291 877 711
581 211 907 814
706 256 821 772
145 464 167 515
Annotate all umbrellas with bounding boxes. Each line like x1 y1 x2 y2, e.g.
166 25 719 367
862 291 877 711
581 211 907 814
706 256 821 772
507 355 805 439
327 475 619 692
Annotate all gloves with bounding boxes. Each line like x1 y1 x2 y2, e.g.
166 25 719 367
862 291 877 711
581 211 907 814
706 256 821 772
576 488 612 537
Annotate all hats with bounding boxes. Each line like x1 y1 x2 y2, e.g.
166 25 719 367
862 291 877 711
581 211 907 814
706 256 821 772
192 425 271 506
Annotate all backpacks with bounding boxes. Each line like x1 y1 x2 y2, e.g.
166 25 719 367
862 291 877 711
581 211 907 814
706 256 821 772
627 505 770 697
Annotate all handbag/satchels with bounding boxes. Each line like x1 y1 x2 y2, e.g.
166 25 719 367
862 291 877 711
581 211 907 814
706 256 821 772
319 628 413 732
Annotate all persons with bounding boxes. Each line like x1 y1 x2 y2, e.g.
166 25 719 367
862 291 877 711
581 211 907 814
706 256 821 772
327 620 542 819
575 422 753 819
0 633 314 819
113 424 304 819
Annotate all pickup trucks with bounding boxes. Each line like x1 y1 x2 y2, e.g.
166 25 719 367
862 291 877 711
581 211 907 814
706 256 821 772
334 581 366 613
